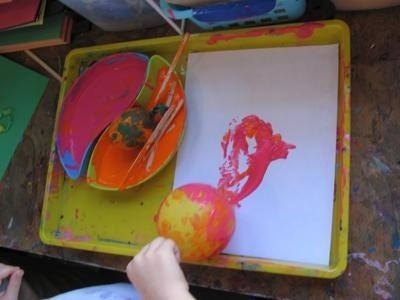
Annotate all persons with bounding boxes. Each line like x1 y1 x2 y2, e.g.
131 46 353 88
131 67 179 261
0 236 196 299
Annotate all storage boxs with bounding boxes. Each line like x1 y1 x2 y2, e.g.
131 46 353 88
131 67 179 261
60 0 162 31
160 0 306 31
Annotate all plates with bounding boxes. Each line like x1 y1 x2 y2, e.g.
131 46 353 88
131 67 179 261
86 54 187 192
39 19 351 279
54 53 149 182
